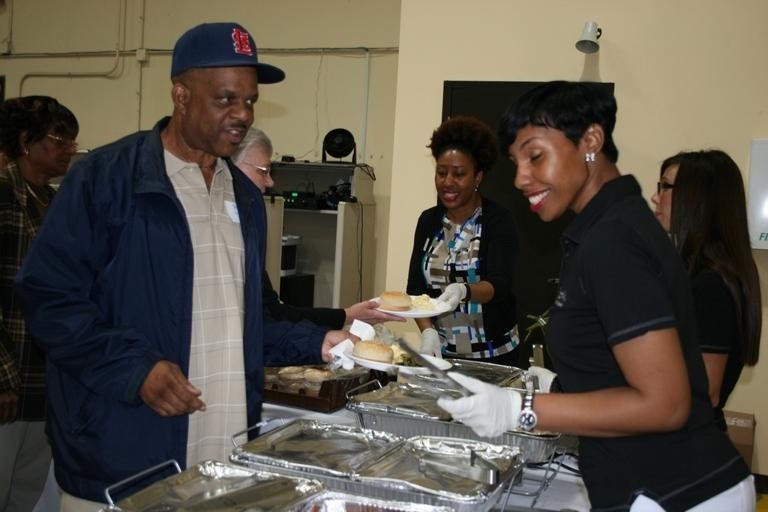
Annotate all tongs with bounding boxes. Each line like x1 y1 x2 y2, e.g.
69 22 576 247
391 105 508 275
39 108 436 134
385 337 473 398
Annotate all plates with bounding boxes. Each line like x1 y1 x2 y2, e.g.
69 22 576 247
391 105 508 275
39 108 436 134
371 295 452 318
344 348 452 376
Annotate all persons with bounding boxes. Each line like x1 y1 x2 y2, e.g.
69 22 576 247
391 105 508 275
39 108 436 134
405 116 521 369
0 95 80 512
231 128 406 331
437 79 759 512
16 21 362 512
652 146 763 440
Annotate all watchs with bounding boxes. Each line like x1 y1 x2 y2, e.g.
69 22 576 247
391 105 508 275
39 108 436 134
516 392 537 433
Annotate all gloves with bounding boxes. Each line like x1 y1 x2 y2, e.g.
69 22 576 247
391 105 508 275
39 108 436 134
419 328 442 358
436 371 523 438
525 365 558 393
437 282 467 312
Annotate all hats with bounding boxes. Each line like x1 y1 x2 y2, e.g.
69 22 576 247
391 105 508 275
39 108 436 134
170 21 286 84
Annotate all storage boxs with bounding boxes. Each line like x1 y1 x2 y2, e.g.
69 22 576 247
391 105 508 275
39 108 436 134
722 410 756 473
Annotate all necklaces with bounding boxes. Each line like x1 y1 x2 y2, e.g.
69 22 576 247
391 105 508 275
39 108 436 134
24 179 53 207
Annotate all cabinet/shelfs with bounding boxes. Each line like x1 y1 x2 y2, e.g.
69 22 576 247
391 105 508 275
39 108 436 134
264 159 374 308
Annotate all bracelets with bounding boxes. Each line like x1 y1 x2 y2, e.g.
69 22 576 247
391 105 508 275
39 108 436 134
460 280 473 305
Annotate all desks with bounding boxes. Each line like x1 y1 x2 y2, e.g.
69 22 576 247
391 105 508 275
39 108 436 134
102 403 591 511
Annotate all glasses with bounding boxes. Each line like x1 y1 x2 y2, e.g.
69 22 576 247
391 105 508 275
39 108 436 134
657 182 674 194
243 160 271 176
46 134 79 151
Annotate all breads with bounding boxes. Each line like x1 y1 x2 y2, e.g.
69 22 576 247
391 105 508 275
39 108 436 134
377 290 413 311
353 340 394 364
